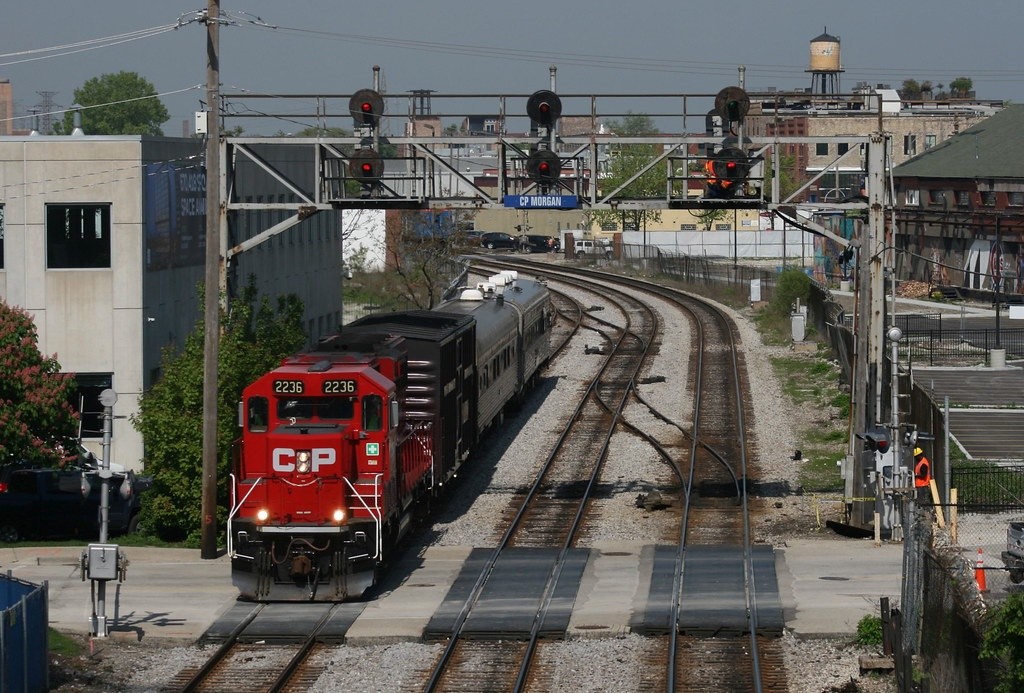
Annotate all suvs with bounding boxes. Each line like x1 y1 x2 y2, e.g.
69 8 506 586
479 232 520 249
574 236 615 259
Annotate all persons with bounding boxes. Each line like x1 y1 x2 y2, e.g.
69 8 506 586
913 447 931 507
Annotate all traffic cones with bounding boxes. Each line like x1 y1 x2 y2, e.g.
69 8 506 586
975 548 987 590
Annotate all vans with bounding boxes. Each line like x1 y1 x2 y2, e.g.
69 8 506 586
520 235 560 253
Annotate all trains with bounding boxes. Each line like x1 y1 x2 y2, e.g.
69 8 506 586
228 271 552 602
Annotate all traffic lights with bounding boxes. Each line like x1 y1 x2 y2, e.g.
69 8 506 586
856 431 891 454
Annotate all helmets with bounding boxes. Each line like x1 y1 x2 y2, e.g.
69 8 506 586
913 447 923 457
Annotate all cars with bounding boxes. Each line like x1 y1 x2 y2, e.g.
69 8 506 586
0 441 172 542
